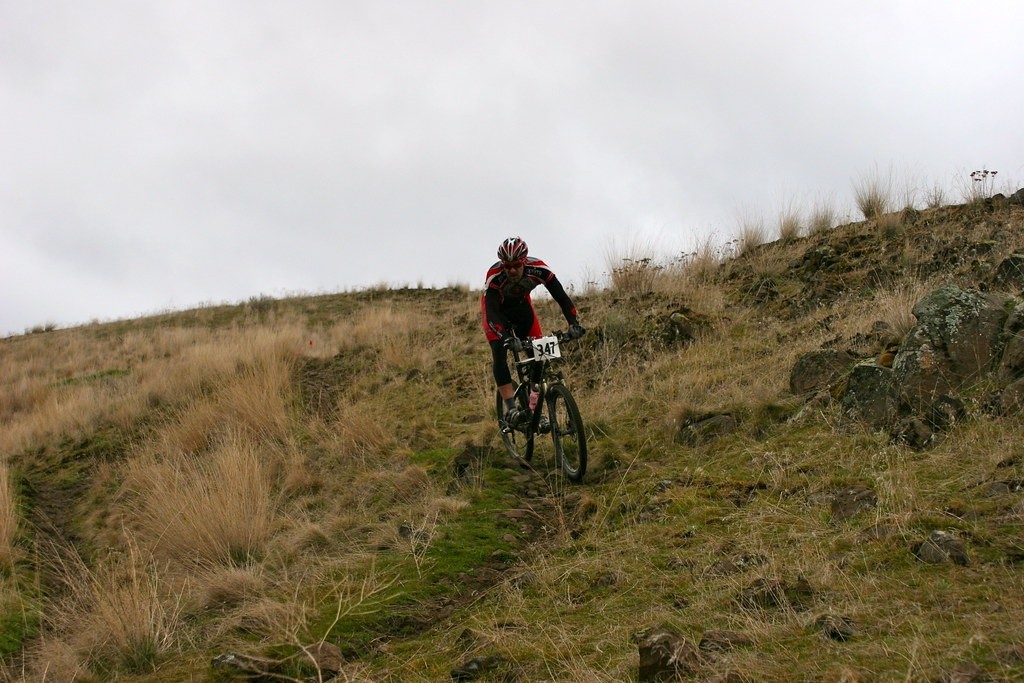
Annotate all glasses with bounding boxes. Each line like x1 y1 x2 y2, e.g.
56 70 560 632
502 257 526 269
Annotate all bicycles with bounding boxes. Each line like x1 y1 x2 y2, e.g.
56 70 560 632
496 328 588 482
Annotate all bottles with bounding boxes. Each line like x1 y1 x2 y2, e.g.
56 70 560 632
529 384 540 409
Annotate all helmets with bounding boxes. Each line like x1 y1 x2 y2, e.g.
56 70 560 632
498 238 528 263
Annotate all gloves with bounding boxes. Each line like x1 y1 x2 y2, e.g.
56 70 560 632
501 336 516 351
567 324 584 340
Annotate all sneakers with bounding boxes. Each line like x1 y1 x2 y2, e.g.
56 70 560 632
506 409 525 425
532 411 551 435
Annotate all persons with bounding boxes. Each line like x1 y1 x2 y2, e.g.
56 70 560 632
482 238 587 434
